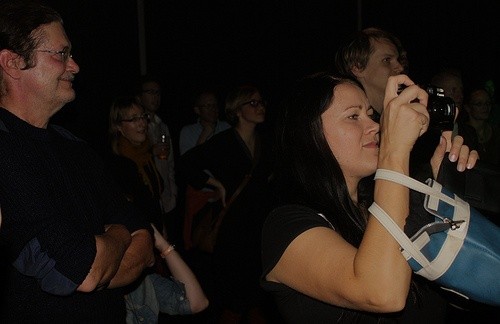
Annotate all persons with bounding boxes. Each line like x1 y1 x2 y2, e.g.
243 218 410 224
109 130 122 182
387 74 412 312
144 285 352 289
183 84 280 308
415 68 500 200
180 93 225 154
134 79 178 240
122 224 209 324
257 73 480 324
0 0 155 324
104 95 164 233
335 27 405 124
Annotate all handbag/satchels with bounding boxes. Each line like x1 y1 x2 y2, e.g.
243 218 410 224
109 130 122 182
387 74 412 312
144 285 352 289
368 169 500 305
192 205 227 253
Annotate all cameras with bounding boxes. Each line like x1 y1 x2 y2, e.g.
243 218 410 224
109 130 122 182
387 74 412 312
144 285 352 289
397 86 460 130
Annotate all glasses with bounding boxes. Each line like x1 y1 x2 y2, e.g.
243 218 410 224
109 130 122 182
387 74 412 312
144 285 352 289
473 101 492 107
200 103 219 108
121 113 148 122
239 100 266 108
32 49 73 62
142 89 162 96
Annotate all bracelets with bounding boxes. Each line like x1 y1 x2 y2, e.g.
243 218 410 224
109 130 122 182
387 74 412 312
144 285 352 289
161 244 175 258
